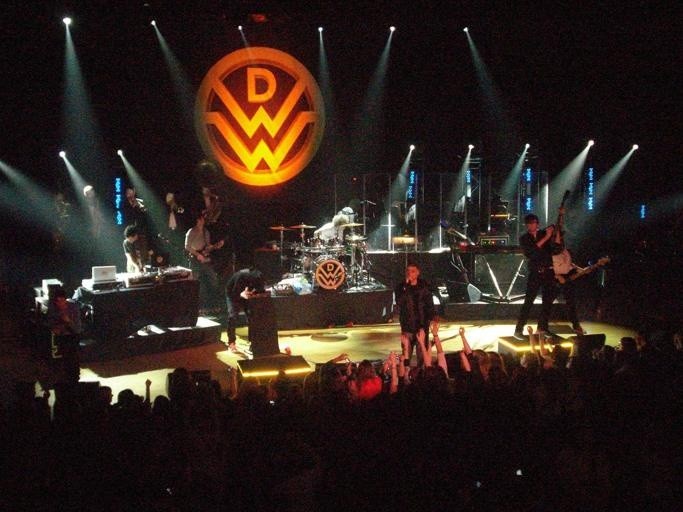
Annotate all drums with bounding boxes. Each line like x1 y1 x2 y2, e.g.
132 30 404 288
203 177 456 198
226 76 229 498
310 255 344 290
327 247 344 258
302 249 322 273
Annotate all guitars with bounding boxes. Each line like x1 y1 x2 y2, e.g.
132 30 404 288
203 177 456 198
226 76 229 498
543 190 569 255
554 257 612 287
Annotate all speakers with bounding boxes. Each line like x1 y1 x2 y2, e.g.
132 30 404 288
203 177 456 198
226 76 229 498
447 280 482 302
567 334 605 356
167 370 211 399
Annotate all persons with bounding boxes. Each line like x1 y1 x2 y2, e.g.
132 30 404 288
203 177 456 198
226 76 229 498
183 214 225 316
45 183 224 282
550 223 586 337
300 188 457 268
394 263 434 362
517 211 564 338
0 328 683 511
47 290 85 384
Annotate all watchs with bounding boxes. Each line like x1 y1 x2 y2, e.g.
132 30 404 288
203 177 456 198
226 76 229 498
222 267 268 348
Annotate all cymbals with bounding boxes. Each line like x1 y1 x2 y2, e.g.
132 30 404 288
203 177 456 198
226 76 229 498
268 226 289 232
339 222 362 228
290 225 316 229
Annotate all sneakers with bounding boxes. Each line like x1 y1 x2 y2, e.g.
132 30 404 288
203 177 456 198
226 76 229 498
514 332 528 341
576 325 583 334
230 342 237 353
543 329 553 338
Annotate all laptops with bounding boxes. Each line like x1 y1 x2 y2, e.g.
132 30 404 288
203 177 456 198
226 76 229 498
92 266 116 282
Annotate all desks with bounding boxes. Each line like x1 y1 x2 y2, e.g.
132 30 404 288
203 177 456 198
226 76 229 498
77 278 200 340
